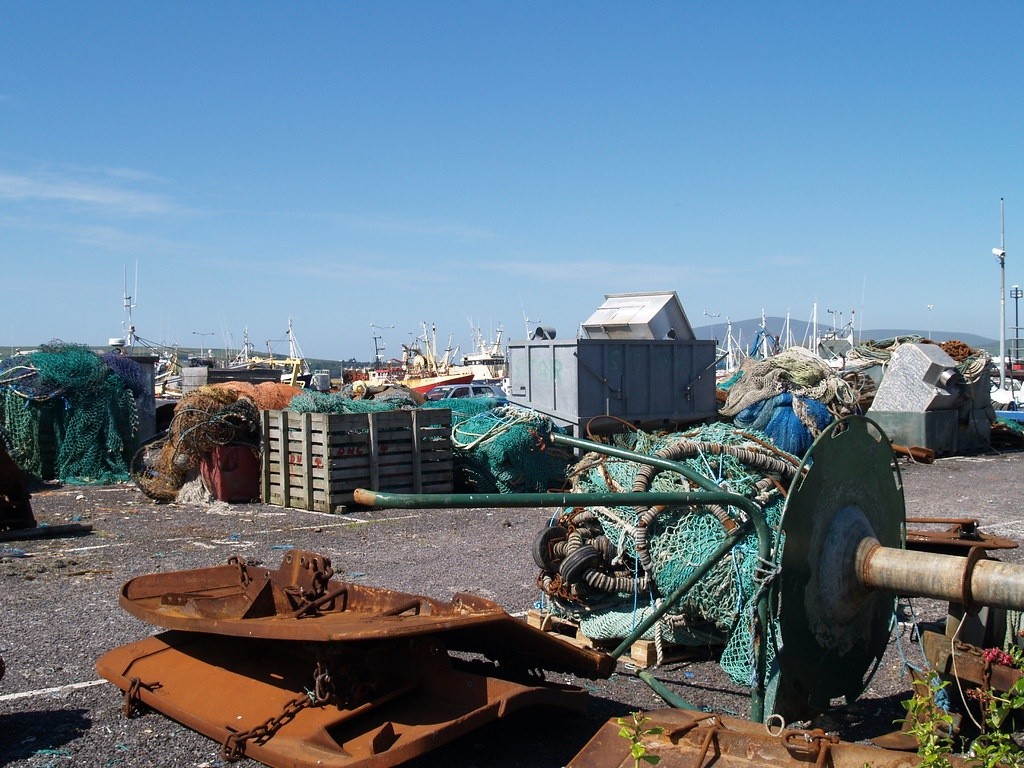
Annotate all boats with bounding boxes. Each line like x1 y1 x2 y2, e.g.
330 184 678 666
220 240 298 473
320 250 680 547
95 253 873 402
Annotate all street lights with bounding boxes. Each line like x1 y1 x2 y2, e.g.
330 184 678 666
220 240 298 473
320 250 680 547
1011 285 1019 365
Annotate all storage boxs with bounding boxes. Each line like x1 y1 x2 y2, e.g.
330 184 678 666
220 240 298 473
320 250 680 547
510 339 717 426
584 290 697 340
